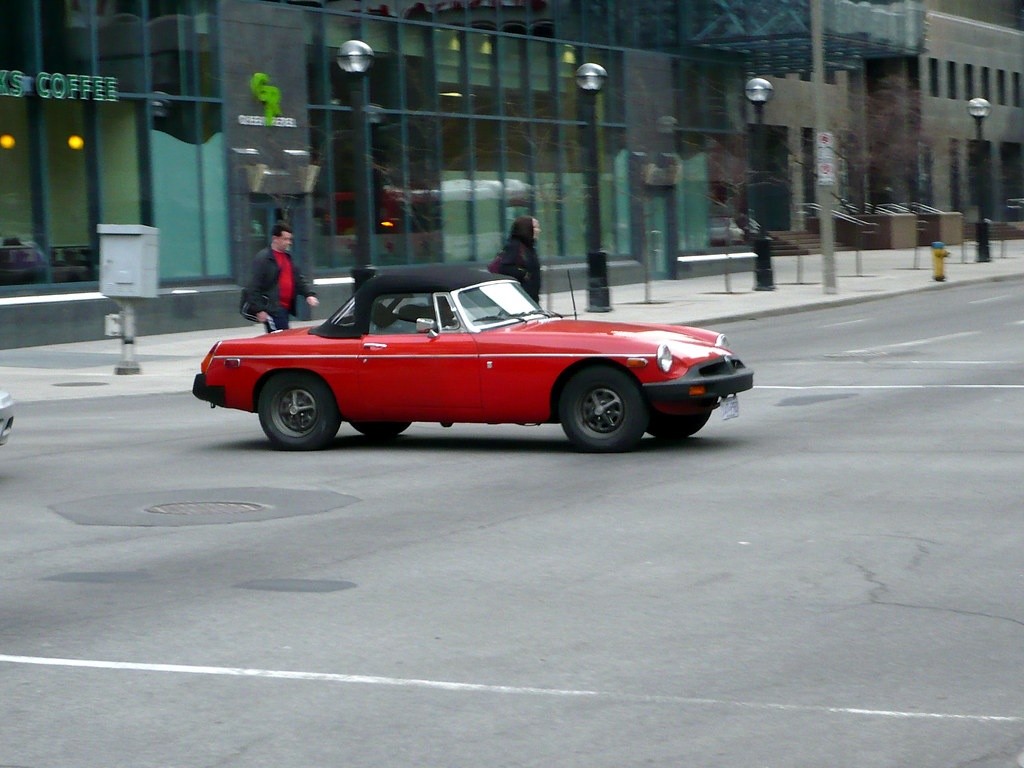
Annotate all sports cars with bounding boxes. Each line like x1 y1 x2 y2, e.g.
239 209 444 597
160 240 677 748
192 266 756 454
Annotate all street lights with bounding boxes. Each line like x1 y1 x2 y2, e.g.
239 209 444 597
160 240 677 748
575 63 613 315
744 78 774 292
968 98 992 262
335 39 378 291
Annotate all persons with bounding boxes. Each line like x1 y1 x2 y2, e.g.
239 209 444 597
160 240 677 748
240 221 320 332
487 215 542 306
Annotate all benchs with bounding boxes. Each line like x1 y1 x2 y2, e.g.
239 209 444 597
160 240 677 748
397 304 436 323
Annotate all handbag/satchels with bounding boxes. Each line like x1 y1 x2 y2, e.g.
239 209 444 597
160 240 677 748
487 242 526 276
238 288 271 322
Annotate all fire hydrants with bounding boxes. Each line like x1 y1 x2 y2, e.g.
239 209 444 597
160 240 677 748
930 239 952 283
